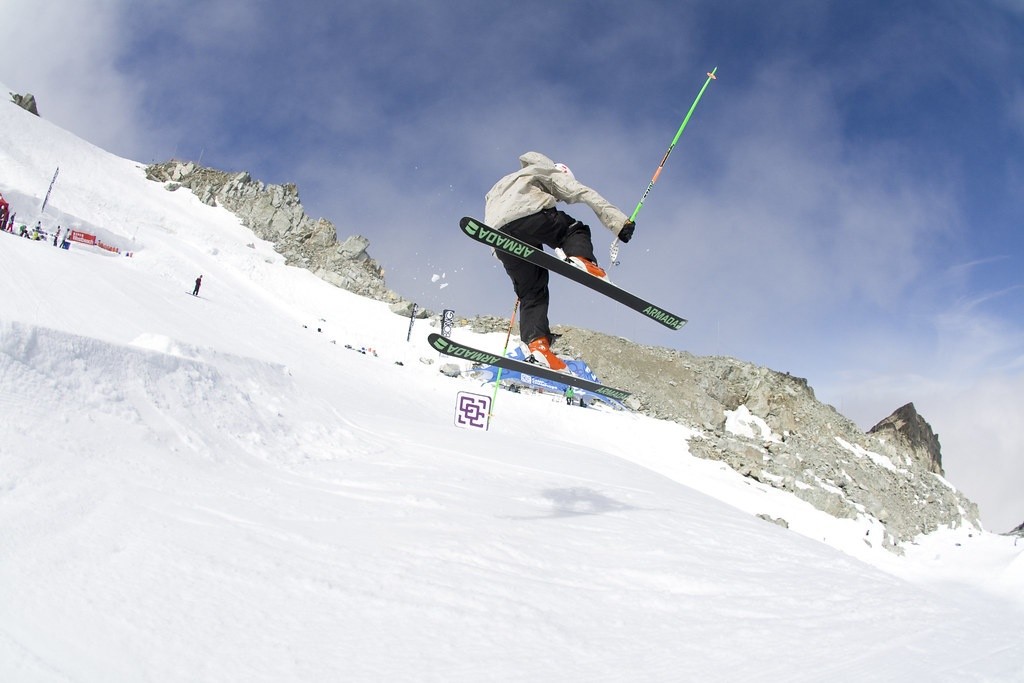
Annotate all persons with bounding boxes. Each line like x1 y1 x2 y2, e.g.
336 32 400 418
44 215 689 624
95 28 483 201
193 274 203 296
2 211 71 248
484 151 636 375
566 386 574 405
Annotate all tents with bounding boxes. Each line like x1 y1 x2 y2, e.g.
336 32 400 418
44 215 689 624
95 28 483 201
0 193 11 231
457 343 640 424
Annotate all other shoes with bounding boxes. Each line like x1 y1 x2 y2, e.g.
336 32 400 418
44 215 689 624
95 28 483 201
525 338 568 374
567 255 607 279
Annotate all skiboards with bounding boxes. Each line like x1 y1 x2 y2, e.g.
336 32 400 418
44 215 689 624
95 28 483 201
427 216 688 402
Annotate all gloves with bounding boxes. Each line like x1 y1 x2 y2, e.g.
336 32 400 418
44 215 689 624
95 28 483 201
618 221 636 244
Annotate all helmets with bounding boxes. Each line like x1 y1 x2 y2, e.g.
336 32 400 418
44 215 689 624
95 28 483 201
553 162 576 181
